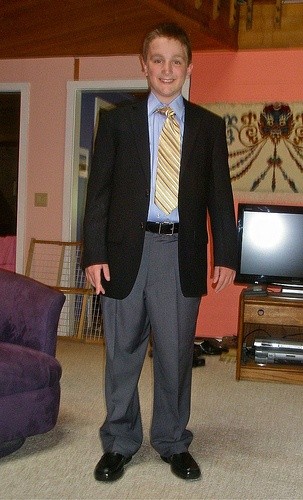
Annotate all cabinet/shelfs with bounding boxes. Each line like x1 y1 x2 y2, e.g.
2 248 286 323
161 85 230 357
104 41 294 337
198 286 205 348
233 290 303 383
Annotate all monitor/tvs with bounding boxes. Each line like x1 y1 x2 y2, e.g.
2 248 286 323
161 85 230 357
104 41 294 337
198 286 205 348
235 203 303 285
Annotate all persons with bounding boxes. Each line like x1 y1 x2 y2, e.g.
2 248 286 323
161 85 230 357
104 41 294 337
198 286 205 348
79 22 238 482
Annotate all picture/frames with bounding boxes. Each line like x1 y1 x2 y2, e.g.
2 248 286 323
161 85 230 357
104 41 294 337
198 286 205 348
79 148 89 178
94 96 118 152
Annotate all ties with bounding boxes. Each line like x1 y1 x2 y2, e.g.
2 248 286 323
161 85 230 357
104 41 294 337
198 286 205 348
154 107 180 215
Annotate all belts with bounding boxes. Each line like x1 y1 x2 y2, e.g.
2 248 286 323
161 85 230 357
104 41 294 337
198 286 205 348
145 221 179 236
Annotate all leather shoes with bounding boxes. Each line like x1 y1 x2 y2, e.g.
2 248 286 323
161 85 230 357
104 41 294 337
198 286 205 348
94 452 132 481
160 451 201 479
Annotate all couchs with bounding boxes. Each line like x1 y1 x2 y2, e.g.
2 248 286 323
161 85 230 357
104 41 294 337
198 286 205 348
0 269 67 460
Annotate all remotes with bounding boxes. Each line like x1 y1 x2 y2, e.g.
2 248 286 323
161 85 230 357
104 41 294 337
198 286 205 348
244 290 267 296
